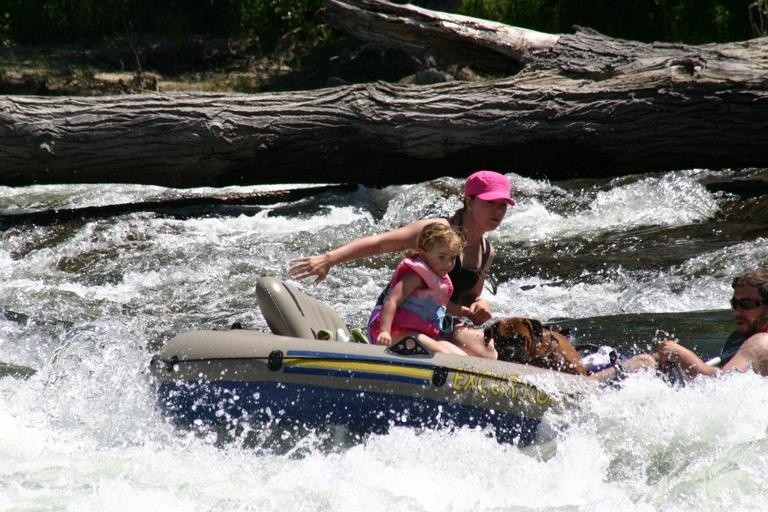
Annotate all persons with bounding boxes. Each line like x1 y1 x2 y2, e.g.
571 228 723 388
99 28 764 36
365 221 470 358
287 169 517 362
586 263 767 390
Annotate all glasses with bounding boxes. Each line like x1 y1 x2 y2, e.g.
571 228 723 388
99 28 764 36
730 296 765 310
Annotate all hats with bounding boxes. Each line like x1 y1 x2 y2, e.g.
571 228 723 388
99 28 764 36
463 170 515 206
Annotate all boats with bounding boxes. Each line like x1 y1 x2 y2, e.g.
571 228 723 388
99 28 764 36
143 272 634 441
